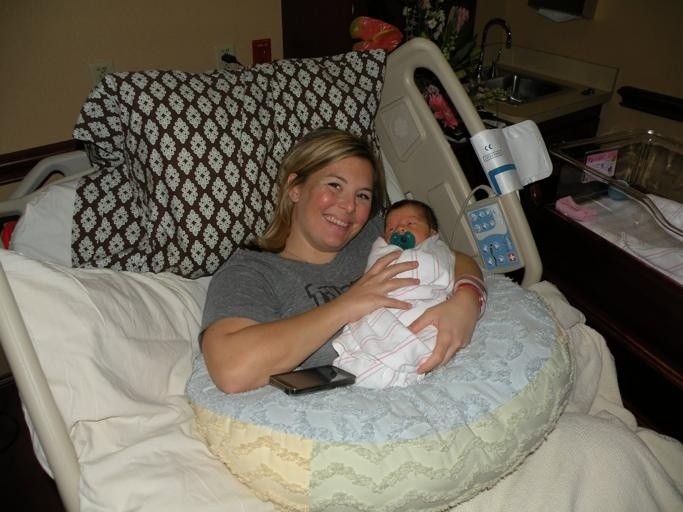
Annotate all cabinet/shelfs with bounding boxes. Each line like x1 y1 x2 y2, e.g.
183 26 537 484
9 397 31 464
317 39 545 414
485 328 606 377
520 130 683 443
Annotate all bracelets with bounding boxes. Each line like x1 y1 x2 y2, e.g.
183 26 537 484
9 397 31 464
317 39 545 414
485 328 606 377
450 275 487 318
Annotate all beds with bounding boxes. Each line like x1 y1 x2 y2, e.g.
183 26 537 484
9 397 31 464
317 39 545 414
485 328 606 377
0 37 683 512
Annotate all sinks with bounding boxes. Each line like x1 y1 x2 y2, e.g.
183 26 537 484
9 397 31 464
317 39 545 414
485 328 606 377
480 69 572 105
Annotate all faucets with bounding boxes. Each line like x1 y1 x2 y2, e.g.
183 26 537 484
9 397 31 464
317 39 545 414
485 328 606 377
475 18 514 83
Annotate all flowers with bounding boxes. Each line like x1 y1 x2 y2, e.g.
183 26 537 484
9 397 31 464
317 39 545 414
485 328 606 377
342 0 509 143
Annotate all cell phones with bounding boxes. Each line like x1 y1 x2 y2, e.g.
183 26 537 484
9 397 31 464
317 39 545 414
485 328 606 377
269 365 356 395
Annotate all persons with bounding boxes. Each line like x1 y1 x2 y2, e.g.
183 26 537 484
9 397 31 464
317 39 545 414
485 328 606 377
382 200 439 254
197 127 485 396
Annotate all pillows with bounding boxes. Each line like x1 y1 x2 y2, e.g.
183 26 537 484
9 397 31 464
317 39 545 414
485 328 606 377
70 48 386 281
